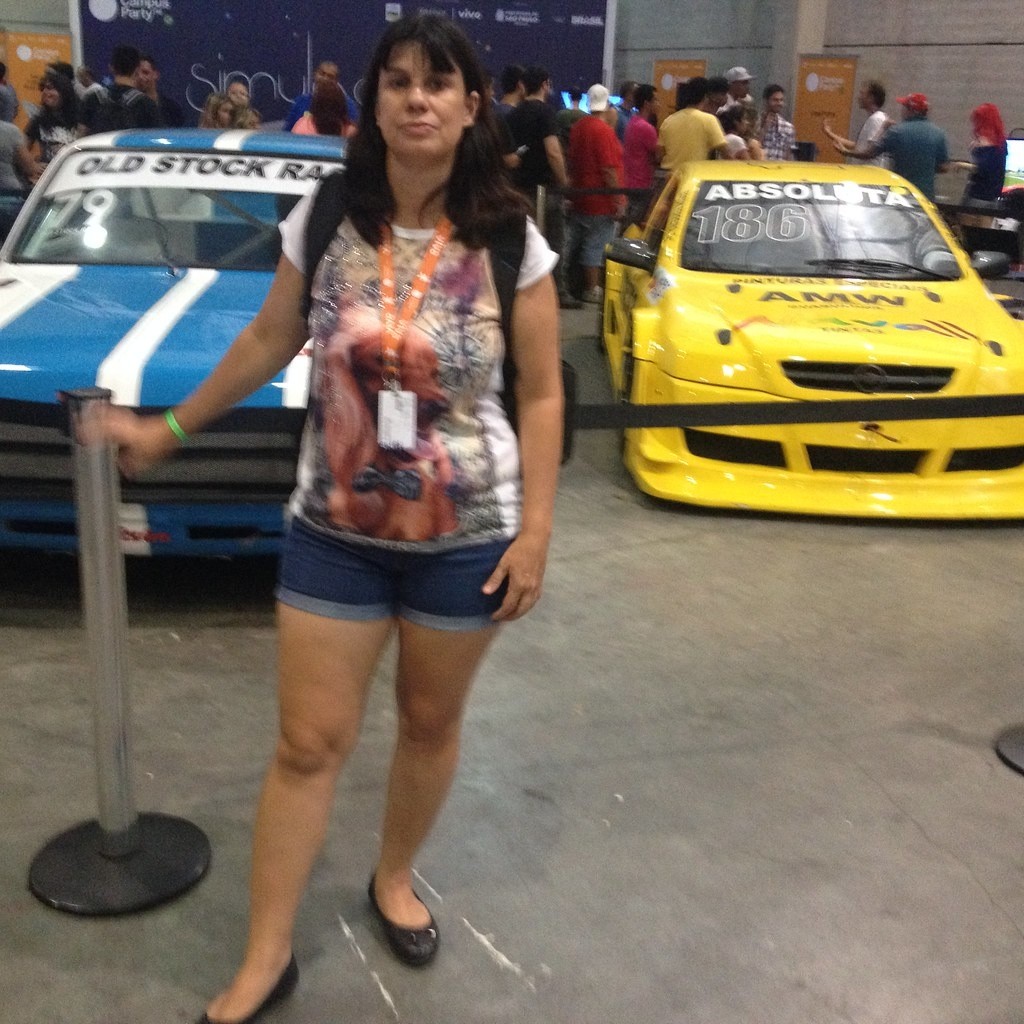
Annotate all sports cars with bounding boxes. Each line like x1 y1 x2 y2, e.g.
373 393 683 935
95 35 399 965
592 154 1024 521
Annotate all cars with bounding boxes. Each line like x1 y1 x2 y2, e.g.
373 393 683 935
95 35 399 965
0 131 353 562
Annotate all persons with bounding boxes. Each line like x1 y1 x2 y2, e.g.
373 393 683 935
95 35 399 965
83 16 561 1024
954 103 1008 227
22 47 182 164
822 82 889 167
478 64 660 308
199 83 259 128
658 77 732 185
276 60 355 221
703 66 798 161
868 92 951 202
0 61 37 224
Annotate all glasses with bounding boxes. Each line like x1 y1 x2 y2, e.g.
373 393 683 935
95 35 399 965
38 83 56 91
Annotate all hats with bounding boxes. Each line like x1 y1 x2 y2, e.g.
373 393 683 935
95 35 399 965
228 74 249 88
896 94 928 116
725 68 754 83
586 83 609 110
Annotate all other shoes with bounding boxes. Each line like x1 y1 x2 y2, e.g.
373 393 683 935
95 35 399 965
559 292 583 310
197 952 298 1024
369 874 440 966
581 285 604 304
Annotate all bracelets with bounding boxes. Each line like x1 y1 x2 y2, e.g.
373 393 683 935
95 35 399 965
165 410 191 446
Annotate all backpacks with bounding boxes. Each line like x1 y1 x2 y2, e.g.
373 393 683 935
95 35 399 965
87 86 145 137
